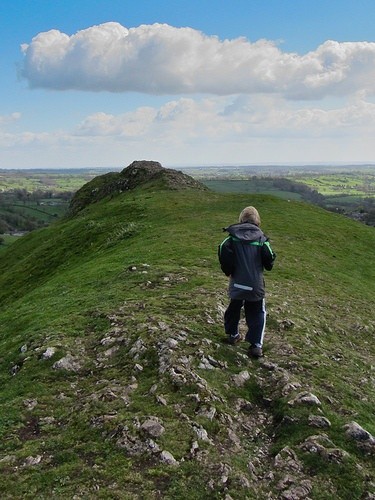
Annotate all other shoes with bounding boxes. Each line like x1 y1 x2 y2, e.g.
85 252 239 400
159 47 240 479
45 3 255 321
229 333 242 344
250 343 264 357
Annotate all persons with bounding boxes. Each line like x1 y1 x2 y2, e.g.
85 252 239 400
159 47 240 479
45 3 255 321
218 206 276 357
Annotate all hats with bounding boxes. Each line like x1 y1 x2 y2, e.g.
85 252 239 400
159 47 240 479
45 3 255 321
238 205 261 225
